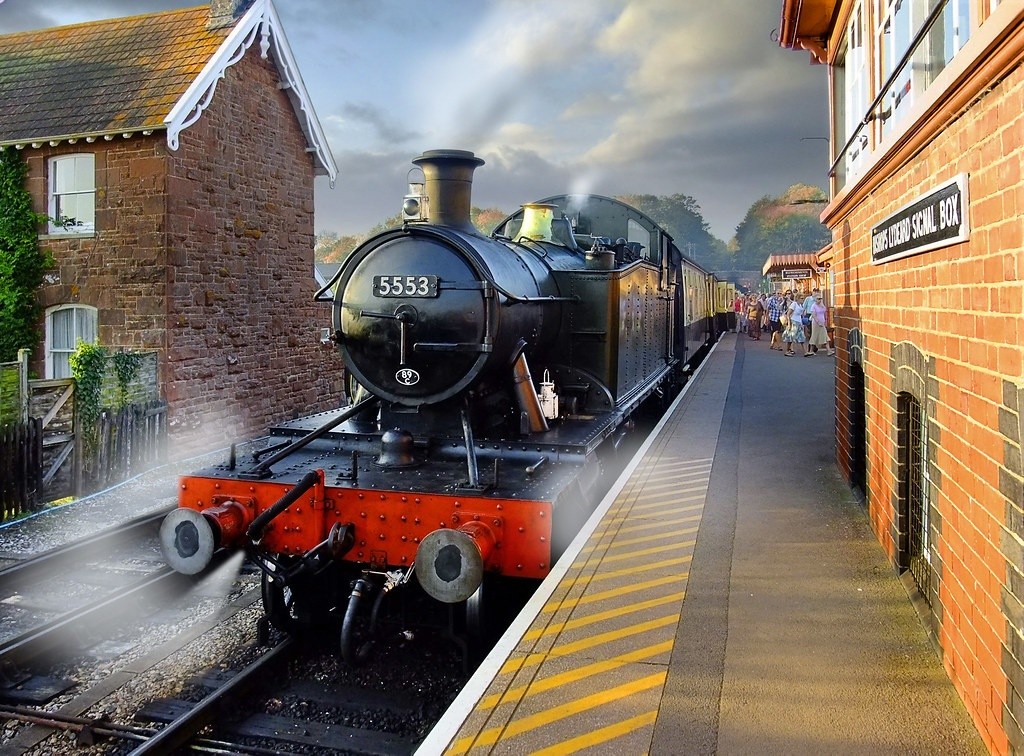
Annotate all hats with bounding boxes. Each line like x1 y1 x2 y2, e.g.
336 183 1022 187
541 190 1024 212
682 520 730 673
813 288 821 292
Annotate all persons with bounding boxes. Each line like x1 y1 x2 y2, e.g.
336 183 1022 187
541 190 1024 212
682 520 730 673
729 288 835 357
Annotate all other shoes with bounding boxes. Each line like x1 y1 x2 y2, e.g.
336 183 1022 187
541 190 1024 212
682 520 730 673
826 351 836 356
775 345 778 350
784 351 794 357
812 352 817 356
753 337 757 341
778 346 783 351
789 349 795 354
770 343 774 349
804 352 814 357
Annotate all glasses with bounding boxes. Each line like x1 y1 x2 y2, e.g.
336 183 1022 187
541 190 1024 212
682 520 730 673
785 292 791 295
799 298 805 299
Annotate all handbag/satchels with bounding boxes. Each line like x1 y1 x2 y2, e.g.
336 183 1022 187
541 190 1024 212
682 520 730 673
779 302 797 326
801 311 810 325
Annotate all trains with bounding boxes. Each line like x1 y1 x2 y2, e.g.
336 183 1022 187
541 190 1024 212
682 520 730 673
159 150 740 665
